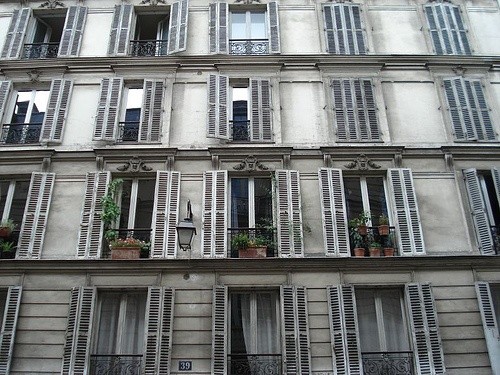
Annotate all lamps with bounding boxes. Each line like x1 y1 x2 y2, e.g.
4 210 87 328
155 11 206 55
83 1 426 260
175 198 197 251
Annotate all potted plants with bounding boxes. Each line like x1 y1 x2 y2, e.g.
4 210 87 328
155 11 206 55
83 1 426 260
384 235 395 257
353 232 365 257
378 213 389 236
0 241 17 259
230 232 275 258
0 219 15 237
368 242 382 257
109 236 144 259
349 211 371 236
